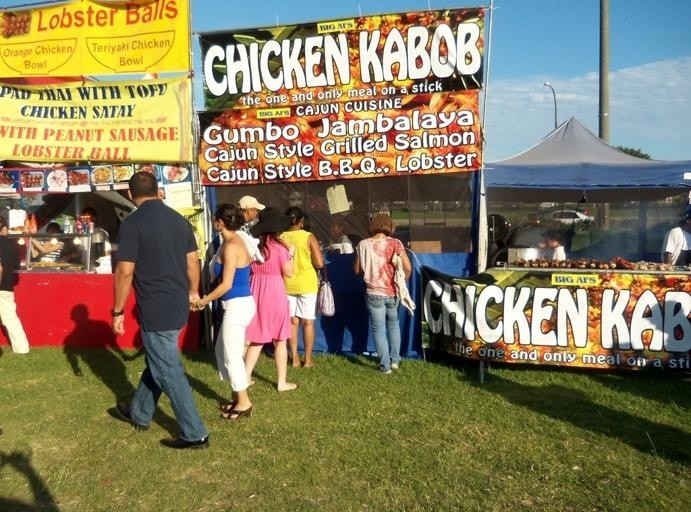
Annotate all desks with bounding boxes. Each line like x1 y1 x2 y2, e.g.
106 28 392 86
0 272 200 352
475 257 691 383
212 249 474 358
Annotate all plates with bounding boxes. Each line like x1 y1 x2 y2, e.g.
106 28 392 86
0 162 189 188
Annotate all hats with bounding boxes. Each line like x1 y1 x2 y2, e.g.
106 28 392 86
249 207 288 238
238 196 266 211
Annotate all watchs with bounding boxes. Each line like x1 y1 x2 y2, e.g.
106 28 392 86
110 308 124 316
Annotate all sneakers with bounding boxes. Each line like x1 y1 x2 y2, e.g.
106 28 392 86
391 362 399 369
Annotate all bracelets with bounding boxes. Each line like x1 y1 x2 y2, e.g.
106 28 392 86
194 299 205 312
290 255 295 260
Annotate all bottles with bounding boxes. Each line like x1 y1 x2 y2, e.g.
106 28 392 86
64 215 94 234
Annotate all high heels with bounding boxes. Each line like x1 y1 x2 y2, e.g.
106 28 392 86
221 406 253 420
220 401 237 412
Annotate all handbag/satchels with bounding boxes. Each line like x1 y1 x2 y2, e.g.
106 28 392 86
391 253 398 267
317 280 336 316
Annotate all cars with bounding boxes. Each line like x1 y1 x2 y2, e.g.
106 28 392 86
400 203 433 212
543 210 594 225
527 206 590 223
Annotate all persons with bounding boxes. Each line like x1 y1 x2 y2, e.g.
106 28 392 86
205 195 268 259
352 212 413 373
1 210 108 368
326 220 353 253
110 170 211 449
192 203 257 420
278 207 324 370
660 208 691 265
238 205 298 392
539 230 566 262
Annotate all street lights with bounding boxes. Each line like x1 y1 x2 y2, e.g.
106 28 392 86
542 81 558 128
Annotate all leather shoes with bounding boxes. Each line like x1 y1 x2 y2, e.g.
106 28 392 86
116 401 151 430
160 436 209 450
